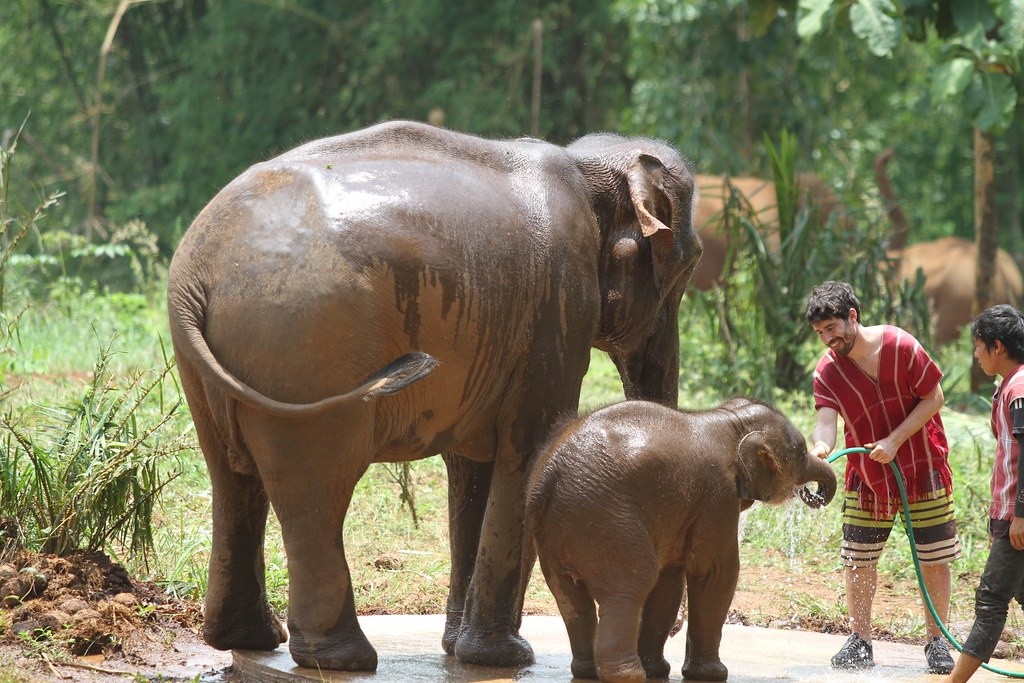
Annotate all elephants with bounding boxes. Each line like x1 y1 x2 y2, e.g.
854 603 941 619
513 396 838 683
168 119 705 675
689 147 1024 357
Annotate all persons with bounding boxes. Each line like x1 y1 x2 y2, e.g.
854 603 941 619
944 304 1024 683
804 280 955 674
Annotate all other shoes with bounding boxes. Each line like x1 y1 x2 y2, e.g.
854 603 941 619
923 636 955 674
830 632 875 669
